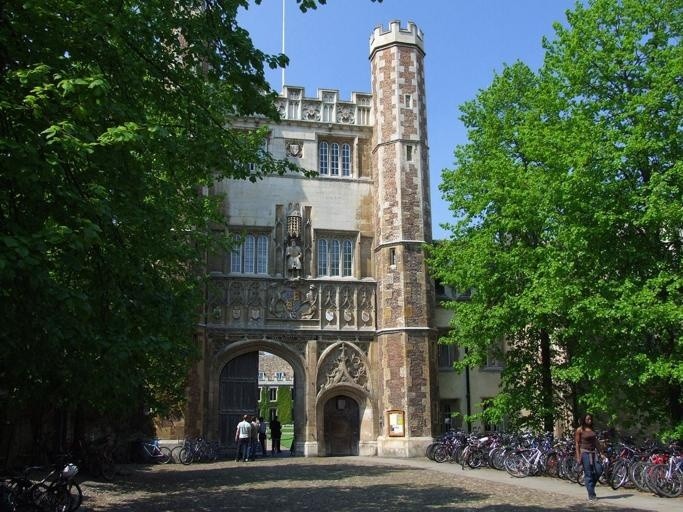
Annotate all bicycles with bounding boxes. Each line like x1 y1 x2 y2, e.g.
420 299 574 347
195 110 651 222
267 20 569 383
425 426 682 499
0 431 218 511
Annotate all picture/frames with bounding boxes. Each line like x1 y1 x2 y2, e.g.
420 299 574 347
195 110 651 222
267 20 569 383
387 409 405 437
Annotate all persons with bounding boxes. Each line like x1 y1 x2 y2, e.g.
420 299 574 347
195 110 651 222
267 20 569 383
260 417 268 455
286 239 302 277
249 417 260 461
270 415 283 455
575 412 610 503
235 415 252 463
